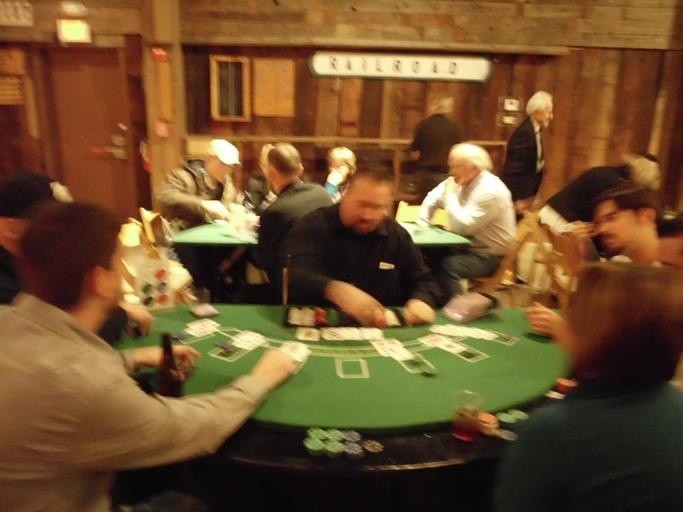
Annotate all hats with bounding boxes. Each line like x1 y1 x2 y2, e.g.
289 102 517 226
206 137 243 169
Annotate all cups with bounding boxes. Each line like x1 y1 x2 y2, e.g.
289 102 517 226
452 389 483 443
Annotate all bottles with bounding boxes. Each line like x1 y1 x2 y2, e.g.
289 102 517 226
156 333 184 396
529 257 553 341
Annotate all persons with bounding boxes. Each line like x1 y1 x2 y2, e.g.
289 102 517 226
518 182 681 343
245 139 334 285
399 99 466 176
490 259 682 510
235 141 277 213
500 90 553 221
278 158 442 327
0 169 155 350
535 146 661 238
414 142 518 310
0 201 313 511
323 145 356 205
153 137 255 301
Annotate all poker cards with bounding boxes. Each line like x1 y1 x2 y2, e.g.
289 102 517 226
188 302 218 320
294 324 384 345
369 337 416 362
231 329 267 351
184 319 220 339
442 291 493 324
429 323 501 343
416 332 469 356
280 340 311 370
383 308 399 328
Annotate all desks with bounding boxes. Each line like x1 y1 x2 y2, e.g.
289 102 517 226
107 207 575 307
108 307 575 510
